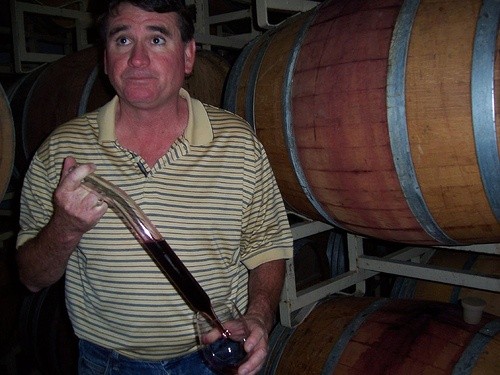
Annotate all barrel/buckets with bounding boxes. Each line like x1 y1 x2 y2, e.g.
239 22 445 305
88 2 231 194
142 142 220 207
388 247 500 315
259 284 500 375
8 43 233 193
222 0 500 246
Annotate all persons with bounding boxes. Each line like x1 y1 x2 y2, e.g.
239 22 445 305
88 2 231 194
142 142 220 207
15 0 294 375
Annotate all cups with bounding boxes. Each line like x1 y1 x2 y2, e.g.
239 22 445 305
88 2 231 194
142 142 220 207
196 300 253 375
